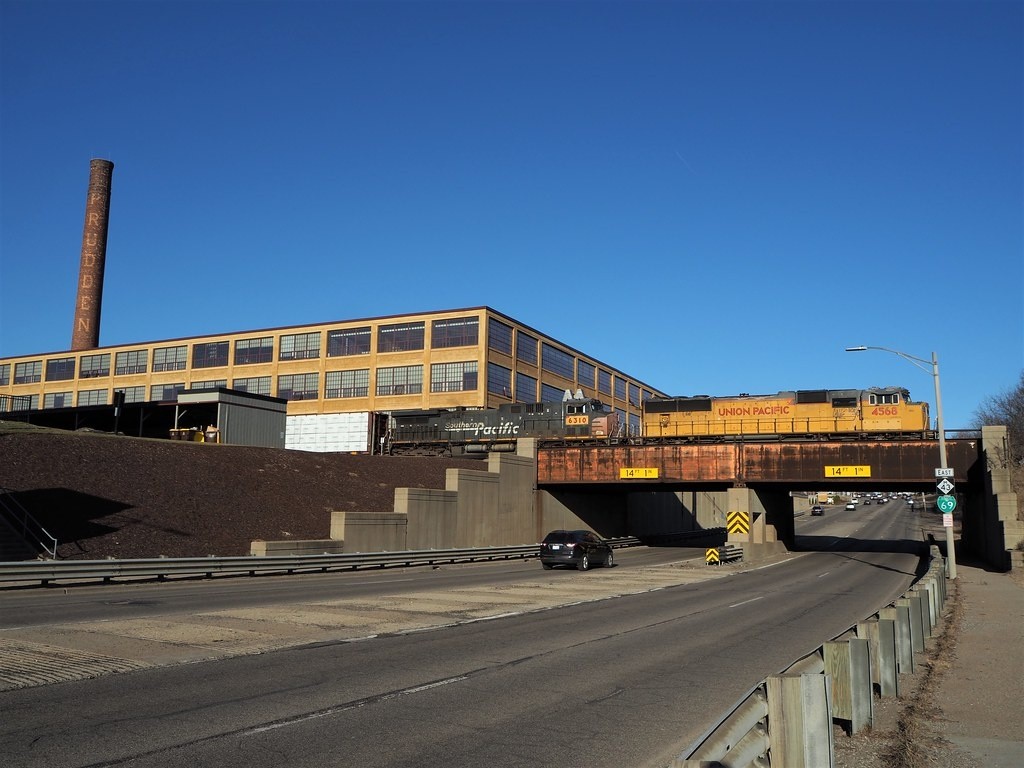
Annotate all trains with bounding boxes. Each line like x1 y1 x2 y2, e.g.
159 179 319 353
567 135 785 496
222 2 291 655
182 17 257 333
285 386 930 458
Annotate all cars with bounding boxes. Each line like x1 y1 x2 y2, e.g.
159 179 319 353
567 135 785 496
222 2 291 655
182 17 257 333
851 498 859 504
864 499 871 505
902 495 914 505
853 492 897 504
846 502 856 511
811 506 825 516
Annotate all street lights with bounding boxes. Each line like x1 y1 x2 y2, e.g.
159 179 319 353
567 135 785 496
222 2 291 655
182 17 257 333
846 346 957 580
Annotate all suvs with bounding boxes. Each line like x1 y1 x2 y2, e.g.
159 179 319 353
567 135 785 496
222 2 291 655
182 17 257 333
540 529 613 571
